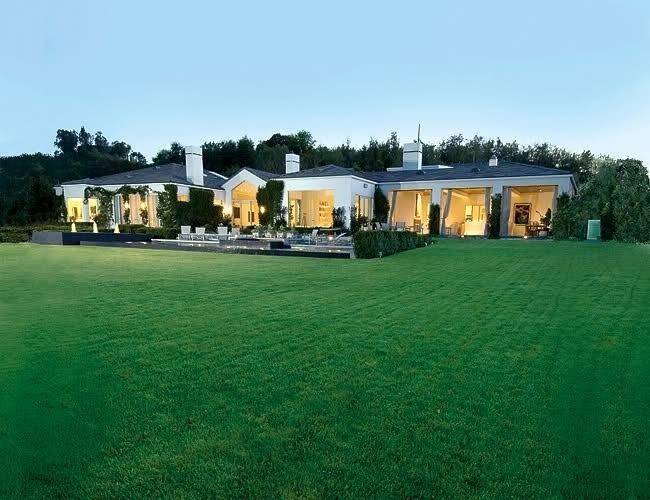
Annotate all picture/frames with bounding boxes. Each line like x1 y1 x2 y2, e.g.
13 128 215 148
513 203 532 226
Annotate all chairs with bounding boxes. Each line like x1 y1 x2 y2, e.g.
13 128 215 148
215 227 228 242
375 219 424 235
326 232 346 245
302 229 319 245
194 227 206 240
230 227 242 239
178 225 193 242
